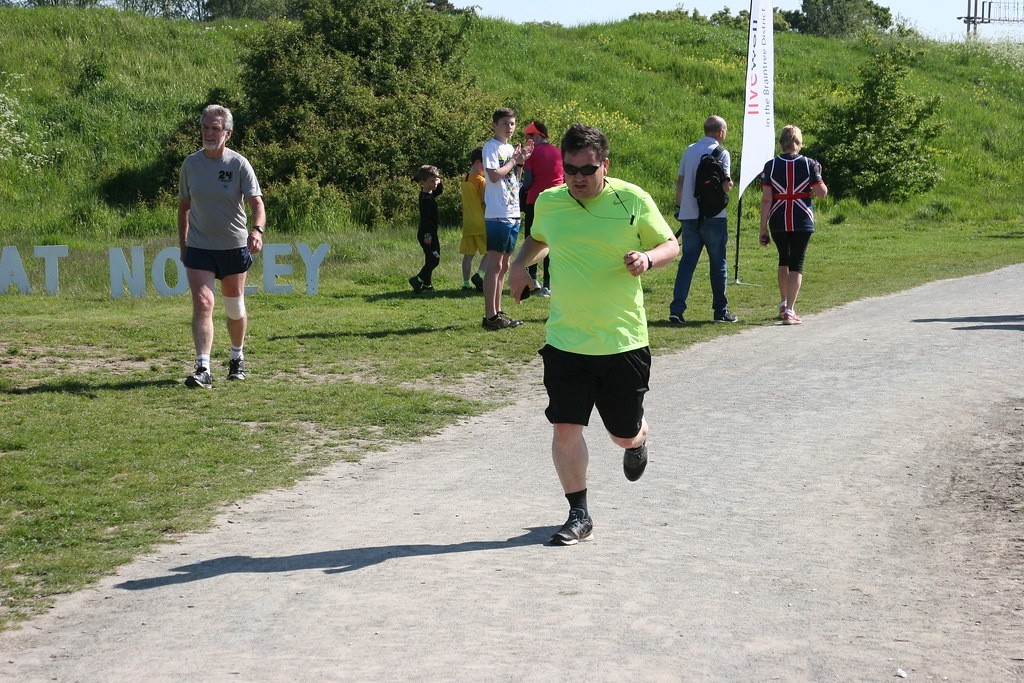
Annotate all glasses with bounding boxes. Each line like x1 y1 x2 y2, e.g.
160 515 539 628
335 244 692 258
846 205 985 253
562 161 602 175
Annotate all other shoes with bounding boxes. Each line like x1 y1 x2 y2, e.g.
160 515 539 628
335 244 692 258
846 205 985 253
530 279 541 292
539 286 550 297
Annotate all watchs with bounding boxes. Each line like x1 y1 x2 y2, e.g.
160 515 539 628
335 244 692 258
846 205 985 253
254 225 264 234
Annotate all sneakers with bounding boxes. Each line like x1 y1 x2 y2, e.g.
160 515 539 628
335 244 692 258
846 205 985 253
462 285 475 293
779 306 788 318
481 313 518 330
228 358 245 381
422 284 434 292
185 366 212 389
782 313 803 325
409 275 425 294
715 313 738 324
622 441 648 482
670 312 685 323
497 311 523 327
471 273 483 291
550 509 595 545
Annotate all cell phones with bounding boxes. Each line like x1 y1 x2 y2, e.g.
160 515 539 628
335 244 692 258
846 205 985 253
520 267 530 300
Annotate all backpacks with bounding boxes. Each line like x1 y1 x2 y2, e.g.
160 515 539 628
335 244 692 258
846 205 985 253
694 146 730 217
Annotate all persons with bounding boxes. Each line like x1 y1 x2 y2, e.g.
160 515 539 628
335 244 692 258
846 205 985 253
177 104 266 387
669 116 737 323
460 147 489 291
507 125 680 544
521 120 563 300
482 108 534 330
759 125 828 325
408 164 445 294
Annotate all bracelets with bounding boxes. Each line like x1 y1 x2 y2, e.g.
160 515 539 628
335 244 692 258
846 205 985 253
644 252 652 270
517 164 524 167
